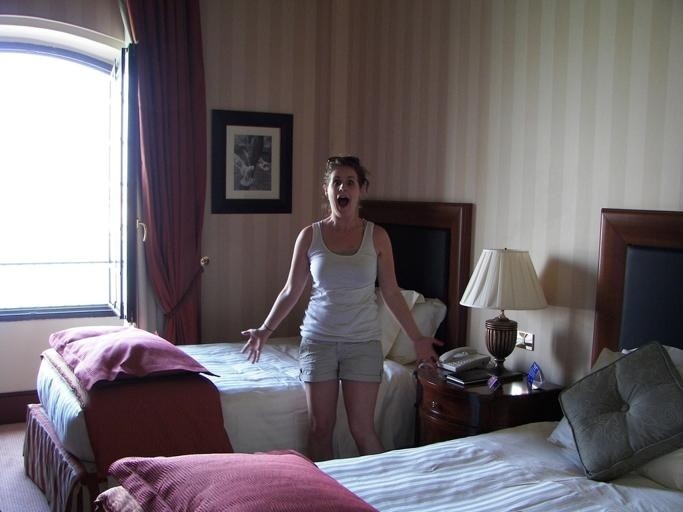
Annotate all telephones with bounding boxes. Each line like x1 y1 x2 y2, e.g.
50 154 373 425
436 347 491 372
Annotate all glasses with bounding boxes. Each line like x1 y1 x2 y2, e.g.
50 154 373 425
324 156 360 175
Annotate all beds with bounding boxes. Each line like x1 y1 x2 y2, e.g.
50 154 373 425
93 204 681 512
22 199 476 510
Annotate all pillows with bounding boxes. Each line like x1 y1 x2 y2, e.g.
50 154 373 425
108 448 380 512
373 285 447 368
544 340 681 495
48 323 219 392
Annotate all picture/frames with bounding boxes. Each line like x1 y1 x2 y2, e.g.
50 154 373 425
206 106 297 216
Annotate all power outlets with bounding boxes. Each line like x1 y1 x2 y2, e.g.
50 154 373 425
514 330 535 351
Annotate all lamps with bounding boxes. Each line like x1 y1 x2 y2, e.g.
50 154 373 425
458 244 550 384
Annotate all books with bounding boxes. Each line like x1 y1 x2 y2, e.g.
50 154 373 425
445 368 490 386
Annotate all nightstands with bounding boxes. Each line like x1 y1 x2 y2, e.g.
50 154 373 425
412 360 564 451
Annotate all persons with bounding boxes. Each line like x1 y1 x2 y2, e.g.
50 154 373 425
237 155 444 461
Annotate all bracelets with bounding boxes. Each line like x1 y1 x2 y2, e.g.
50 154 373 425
263 322 275 333
